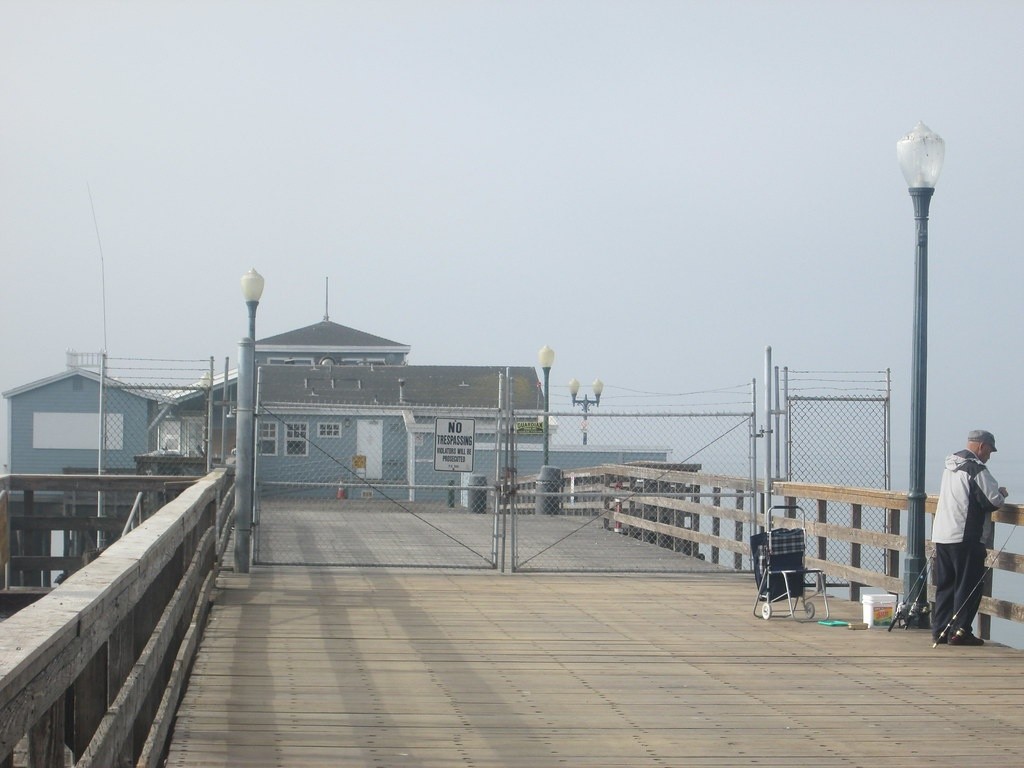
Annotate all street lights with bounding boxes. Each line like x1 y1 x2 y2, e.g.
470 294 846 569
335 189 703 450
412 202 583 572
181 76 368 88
200 371 211 473
895 123 946 629
568 377 604 447
242 268 265 511
539 344 554 466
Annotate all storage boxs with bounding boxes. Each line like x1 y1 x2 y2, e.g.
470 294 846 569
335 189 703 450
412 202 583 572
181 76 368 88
862 593 897 630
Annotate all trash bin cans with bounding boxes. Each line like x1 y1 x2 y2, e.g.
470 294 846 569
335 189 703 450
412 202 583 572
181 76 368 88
535 465 561 516
467 476 487 514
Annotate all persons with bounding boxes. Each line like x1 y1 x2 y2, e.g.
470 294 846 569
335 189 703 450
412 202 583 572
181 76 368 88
932 430 1008 645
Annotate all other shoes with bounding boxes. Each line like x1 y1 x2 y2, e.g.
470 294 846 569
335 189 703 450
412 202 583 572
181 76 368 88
932 627 950 644
948 630 984 646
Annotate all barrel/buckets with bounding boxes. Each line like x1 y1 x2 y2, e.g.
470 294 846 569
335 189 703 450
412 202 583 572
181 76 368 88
862 594 897 629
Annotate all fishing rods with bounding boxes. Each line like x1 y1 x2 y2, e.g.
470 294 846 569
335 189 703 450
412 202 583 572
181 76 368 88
933 505 1024 649
887 547 936 632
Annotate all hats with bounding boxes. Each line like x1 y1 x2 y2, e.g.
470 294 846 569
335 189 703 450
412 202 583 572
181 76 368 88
968 429 998 452
